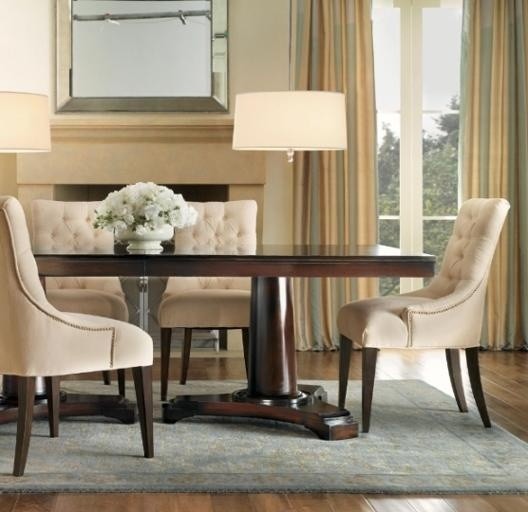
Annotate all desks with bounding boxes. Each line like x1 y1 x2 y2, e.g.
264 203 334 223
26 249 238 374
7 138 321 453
0 243 437 441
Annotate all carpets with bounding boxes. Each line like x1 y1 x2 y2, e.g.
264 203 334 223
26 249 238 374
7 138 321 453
1 377 528 496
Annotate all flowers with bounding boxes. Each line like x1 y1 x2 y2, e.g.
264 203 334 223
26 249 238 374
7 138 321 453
91 180 198 235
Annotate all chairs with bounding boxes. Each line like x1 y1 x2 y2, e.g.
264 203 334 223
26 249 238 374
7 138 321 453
335 196 511 433
156 200 257 402
0 195 156 474
31 196 131 394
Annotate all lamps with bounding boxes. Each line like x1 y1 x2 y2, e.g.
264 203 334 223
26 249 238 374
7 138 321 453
232 0 349 163
0 92 53 153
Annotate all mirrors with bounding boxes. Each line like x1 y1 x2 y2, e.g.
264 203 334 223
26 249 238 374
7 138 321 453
53 0 230 115
